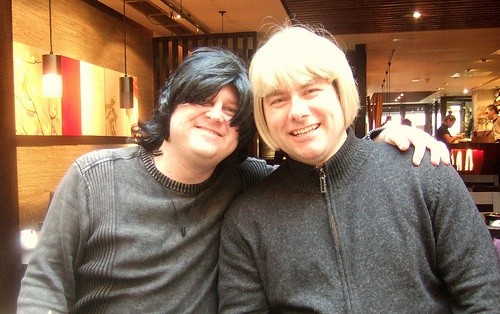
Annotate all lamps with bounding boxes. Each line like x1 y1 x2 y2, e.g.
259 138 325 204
42 0 63 98
118 0 134 109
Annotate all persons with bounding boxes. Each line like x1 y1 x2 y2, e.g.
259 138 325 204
402 118 412 127
16 46 451 314
218 15 500 314
435 111 463 152
485 104 500 142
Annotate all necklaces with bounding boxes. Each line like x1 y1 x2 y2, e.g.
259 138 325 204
165 186 199 236
